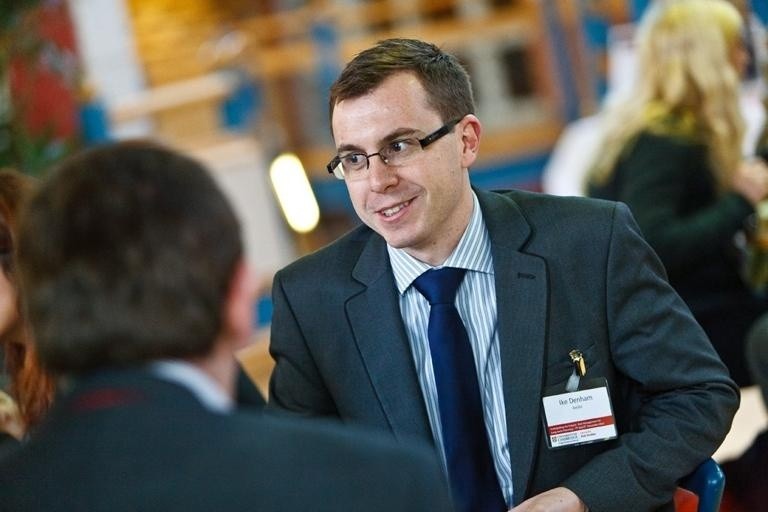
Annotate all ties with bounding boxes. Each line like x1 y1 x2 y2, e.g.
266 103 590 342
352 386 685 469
412 265 510 511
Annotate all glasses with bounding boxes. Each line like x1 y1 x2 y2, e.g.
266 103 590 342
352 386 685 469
325 119 459 181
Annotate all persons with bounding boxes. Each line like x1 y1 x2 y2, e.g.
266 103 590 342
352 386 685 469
1 167 268 436
269 39 740 512
583 0 768 387
0 141 451 512
